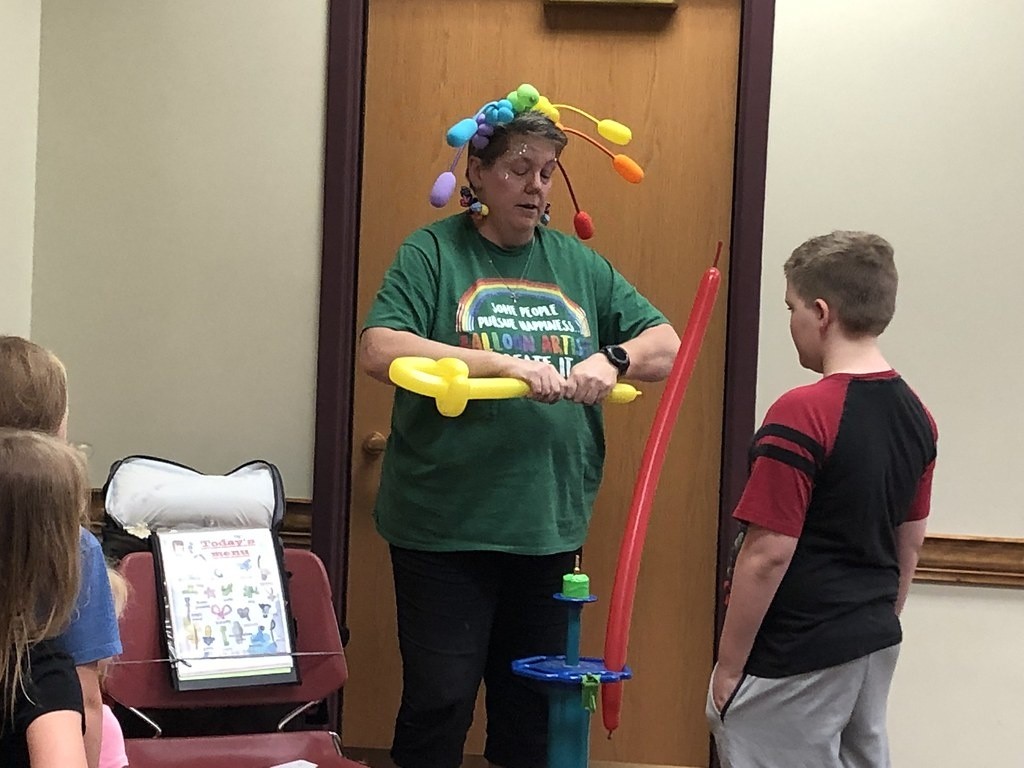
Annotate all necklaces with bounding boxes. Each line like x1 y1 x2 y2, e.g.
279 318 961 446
476 233 535 302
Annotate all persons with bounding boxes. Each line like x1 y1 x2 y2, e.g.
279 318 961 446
0 337 129 768
358 85 680 768
705 228 939 768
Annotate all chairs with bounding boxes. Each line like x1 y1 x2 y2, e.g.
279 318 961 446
96 548 369 768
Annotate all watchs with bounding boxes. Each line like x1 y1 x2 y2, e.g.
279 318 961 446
598 345 629 379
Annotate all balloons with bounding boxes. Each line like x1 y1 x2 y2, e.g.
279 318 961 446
429 82 643 241
388 356 643 417
603 239 722 740
